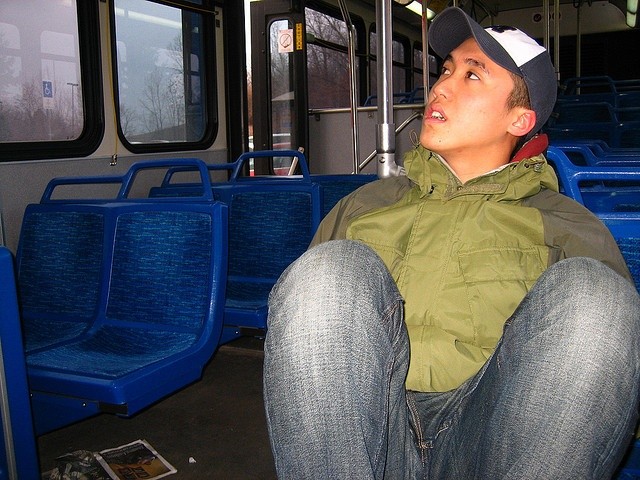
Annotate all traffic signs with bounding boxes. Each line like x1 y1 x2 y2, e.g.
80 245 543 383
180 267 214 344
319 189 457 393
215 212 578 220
43 81 52 98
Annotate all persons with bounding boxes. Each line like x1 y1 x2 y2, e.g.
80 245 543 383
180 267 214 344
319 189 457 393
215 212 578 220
262 8 636 478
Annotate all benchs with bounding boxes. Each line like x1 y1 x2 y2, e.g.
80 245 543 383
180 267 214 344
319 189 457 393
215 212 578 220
1 249 40 479
311 175 377 222
399 97 424 103
365 86 432 106
540 76 639 479
12 158 229 417
149 150 325 330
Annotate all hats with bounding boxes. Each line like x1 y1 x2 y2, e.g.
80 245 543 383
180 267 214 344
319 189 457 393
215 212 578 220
427 7 559 144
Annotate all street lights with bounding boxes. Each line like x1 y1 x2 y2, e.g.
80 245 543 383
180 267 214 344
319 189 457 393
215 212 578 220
66 83 79 139
